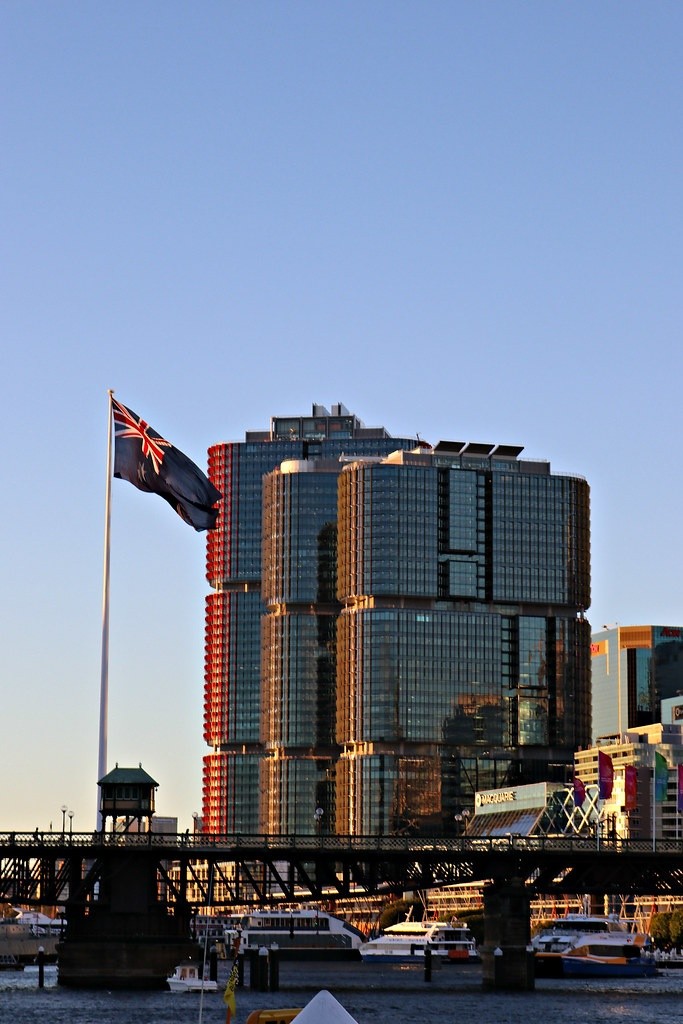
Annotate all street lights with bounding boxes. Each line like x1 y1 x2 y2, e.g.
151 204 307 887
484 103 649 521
61 804 67 843
192 812 199 846
462 808 470 836
316 808 324 835
67 810 74 845
455 814 462 837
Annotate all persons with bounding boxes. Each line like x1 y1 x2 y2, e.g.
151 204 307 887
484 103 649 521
31 827 40 846
185 828 192 848
92 830 97 845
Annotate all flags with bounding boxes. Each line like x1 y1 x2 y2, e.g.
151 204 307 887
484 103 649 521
110 396 224 533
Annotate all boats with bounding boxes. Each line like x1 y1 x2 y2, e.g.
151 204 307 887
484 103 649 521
223 905 370 961
358 905 482 963
165 964 219 992
494 905 660 979
